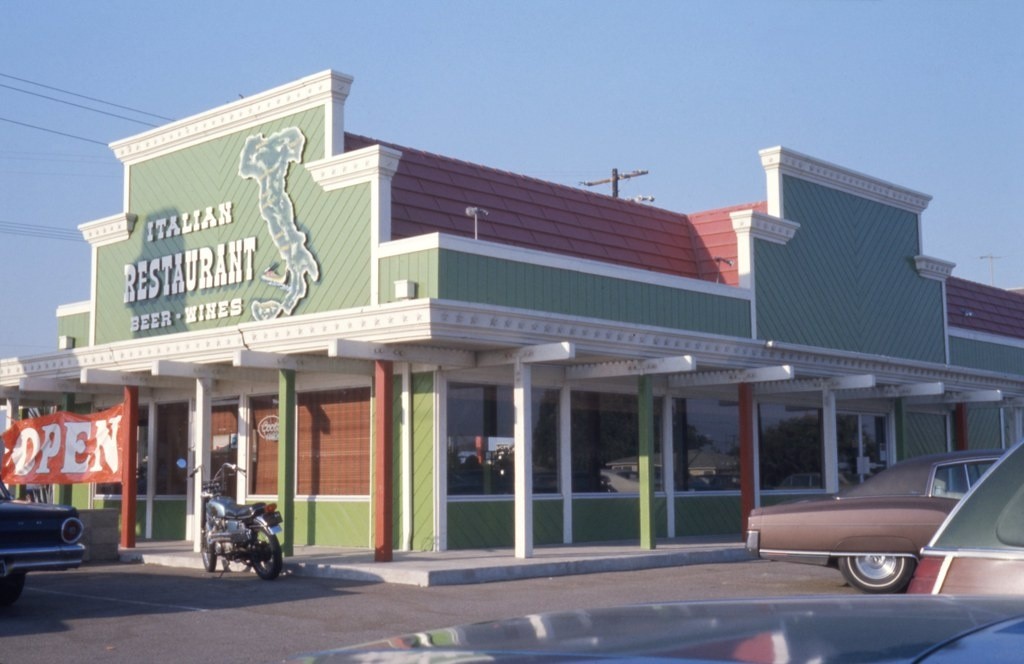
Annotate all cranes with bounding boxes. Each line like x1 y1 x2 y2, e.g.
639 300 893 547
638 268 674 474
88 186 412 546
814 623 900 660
580 167 650 198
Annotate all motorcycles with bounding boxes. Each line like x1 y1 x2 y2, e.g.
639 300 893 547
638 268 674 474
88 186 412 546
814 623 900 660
176 456 284 581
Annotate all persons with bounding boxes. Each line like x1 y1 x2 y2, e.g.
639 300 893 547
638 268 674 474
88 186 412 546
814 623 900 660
26 483 47 503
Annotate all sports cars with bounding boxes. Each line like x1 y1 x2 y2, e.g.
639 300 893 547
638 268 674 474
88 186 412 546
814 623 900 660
744 448 1008 594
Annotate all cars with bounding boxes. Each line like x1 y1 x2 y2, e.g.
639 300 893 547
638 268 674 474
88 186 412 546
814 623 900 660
0 482 85 606
280 593 1024 664
447 442 877 497
